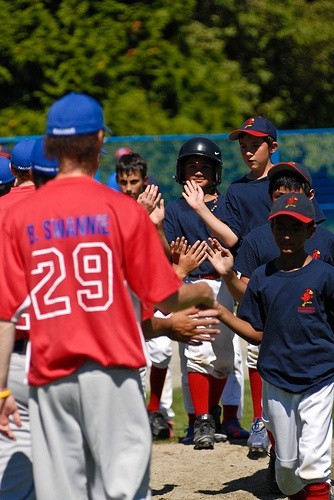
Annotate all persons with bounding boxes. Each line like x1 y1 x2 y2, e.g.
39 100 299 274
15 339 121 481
206 162 334 482
196 193 334 500
142 137 236 446
0 93 220 500
182 116 278 455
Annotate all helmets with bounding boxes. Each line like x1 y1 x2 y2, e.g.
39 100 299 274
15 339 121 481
175 138 222 183
114 147 132 159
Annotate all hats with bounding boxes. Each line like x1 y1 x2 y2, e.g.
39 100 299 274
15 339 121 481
229 117 277 144
267 160 313 191
11 139 37 170
0 156 16 184
101 147 108 153
46 92 113 136
268 194 316 223
31 136 60 176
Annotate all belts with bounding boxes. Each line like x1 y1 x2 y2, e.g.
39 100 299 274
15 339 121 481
188 272 221 280
12 340 24 353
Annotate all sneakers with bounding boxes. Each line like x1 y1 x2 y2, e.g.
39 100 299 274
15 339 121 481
166 418 174 437
147 409 170 440
223 420 250 438
209 405 227 441
247 418 269 452
193 415 215 448
181 413 197 443
